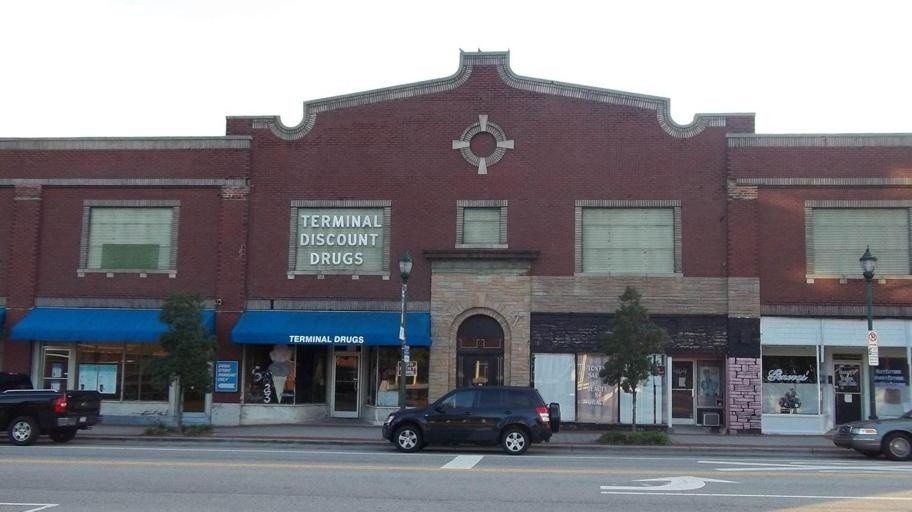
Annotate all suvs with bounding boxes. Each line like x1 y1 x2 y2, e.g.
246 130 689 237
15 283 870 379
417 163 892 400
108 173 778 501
382 386 561 456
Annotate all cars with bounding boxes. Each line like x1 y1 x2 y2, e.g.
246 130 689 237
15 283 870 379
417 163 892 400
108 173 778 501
832 407 912 461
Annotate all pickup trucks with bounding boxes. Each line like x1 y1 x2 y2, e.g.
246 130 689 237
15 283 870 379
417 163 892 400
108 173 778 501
0 370 104 447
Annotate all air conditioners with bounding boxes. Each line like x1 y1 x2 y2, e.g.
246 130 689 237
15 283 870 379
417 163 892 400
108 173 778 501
702 412 720 427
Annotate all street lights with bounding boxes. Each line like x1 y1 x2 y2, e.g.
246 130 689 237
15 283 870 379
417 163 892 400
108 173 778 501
859 245 878 421
397 250 413 411
